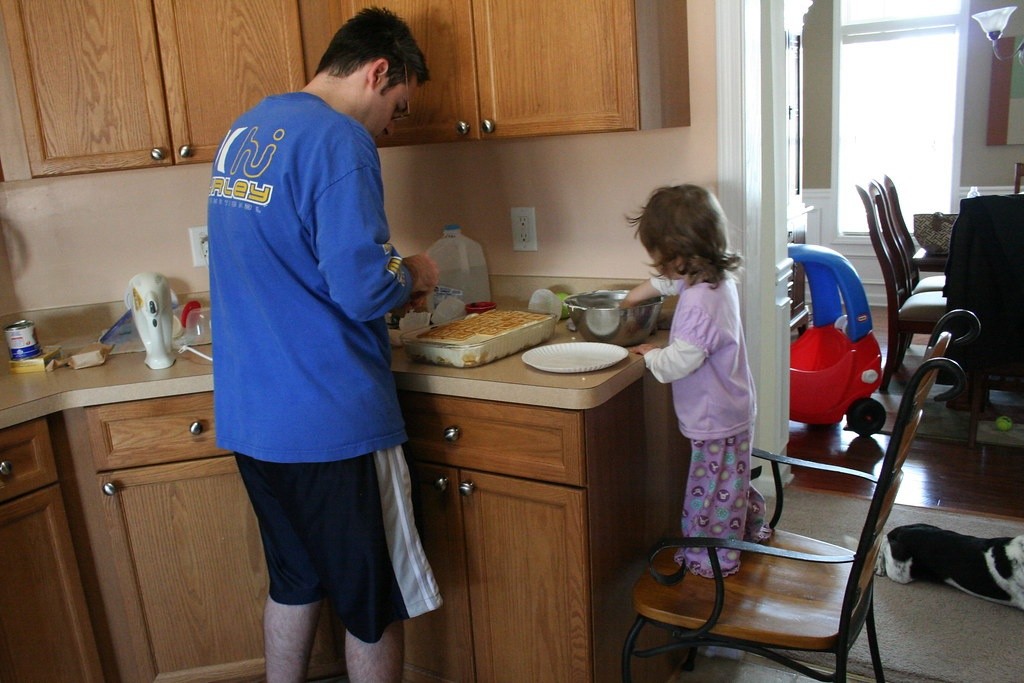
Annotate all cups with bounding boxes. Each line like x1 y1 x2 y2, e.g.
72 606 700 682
185 307 212 345
465 301 498 314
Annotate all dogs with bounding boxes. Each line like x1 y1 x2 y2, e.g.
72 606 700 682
841 524 1024 610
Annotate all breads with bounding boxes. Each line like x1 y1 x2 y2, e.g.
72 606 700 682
417 308 553 343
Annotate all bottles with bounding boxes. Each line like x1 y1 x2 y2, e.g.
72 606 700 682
426 223 491 317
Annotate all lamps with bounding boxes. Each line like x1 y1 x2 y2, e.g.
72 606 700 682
971 6 1024 66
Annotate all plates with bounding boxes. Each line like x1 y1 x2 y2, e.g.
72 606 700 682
522 342 629 372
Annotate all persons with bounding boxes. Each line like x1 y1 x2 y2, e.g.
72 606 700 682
206 7 440 683
620 180 774 578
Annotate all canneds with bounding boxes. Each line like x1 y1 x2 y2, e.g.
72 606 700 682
2 320 42 362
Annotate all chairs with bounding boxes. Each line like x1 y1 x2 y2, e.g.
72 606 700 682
855 162 1024 448
622 308 981 683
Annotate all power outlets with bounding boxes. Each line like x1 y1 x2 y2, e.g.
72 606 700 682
510 207 537 252
188 224 210 267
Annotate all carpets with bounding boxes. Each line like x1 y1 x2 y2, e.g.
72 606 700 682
841 343 1024 449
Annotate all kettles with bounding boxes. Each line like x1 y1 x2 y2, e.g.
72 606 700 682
126 272 177 371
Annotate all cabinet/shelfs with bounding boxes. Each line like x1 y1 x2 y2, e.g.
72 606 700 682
784 27 816 335
0 0 693 185
0 376 685 683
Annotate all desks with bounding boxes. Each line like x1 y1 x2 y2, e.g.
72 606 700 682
911 247 949 273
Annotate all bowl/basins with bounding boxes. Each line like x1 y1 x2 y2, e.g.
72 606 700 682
562 289 665 346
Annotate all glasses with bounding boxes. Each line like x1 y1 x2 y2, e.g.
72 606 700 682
391 63 416 122
